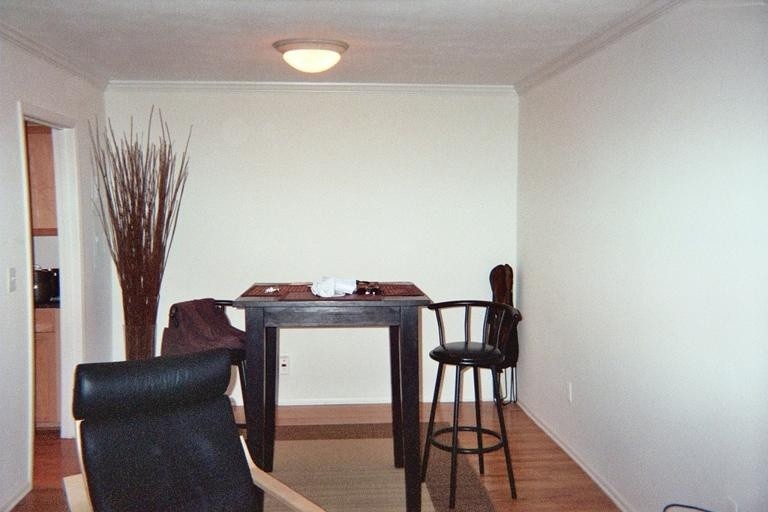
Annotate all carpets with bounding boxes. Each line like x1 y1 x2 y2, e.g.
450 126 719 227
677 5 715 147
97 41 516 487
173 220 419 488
236 422 496 512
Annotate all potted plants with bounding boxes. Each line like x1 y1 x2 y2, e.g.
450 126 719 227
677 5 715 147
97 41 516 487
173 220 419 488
83 104 194 361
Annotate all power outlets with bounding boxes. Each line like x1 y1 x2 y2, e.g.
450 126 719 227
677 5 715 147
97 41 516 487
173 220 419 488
279 356 289 376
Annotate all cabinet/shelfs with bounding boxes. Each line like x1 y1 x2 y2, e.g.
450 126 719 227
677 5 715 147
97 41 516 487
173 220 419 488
24 120 58 237
33 308 61 430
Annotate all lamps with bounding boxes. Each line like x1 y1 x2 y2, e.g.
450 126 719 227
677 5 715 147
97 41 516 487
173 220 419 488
272 36 350 73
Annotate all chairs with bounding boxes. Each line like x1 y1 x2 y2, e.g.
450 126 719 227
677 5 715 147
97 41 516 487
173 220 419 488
422 298 521 498
61 347 325 512
161 297 248 430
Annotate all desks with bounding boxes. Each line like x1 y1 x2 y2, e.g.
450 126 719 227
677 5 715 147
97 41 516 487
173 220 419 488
232 280 434 512
